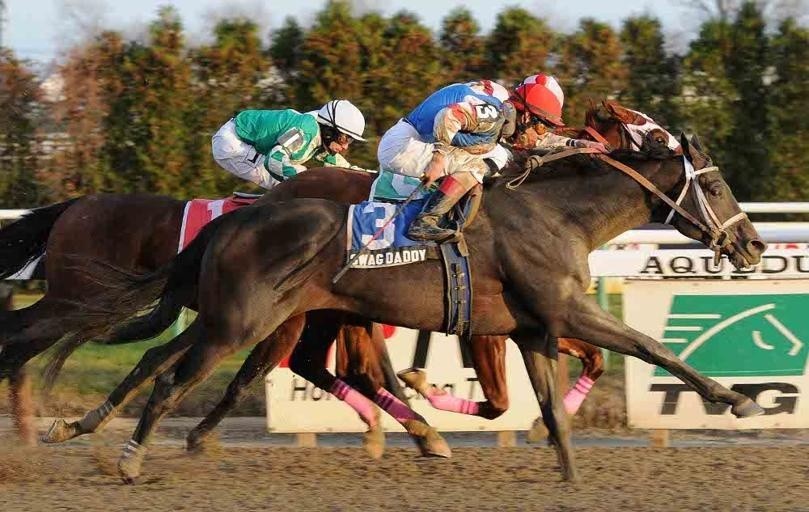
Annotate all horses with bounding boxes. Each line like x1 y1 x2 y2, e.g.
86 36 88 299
37 134 768 490
0 191 307 451
87 96 683 447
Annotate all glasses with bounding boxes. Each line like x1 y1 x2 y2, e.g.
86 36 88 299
335 133 355 144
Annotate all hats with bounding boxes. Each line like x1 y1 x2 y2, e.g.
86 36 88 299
506 84 566 128
317 99 369 142
522 74 564 109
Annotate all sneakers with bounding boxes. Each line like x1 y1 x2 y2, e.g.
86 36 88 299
407 217 455 241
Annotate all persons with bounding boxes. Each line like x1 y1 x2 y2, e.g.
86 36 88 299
378 73 609 243
212 100 379 190
